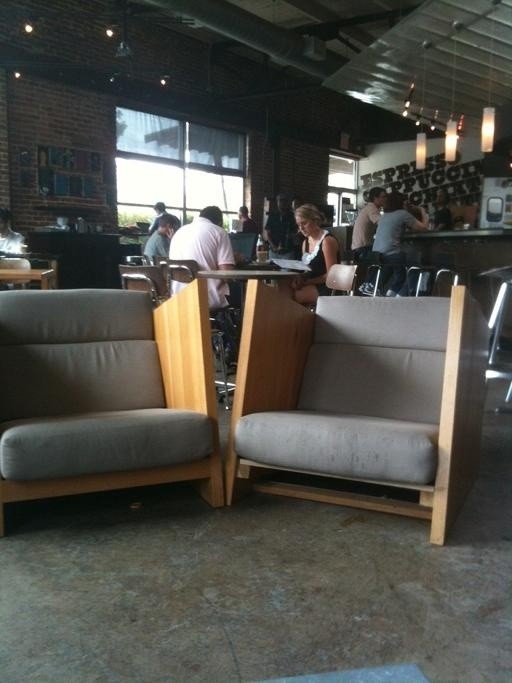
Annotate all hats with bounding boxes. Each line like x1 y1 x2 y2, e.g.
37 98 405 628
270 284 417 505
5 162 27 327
276 193 290 200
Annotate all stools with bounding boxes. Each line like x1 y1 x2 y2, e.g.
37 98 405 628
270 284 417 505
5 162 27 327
478 264 512 365
434 268 455 296
364 250 389 295
407 266 435 297
346 249 363 263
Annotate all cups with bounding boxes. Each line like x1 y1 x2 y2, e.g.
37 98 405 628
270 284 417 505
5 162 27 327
74 217 89 234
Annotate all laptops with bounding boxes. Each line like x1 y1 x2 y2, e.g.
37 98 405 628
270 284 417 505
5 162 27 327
228 231 258 266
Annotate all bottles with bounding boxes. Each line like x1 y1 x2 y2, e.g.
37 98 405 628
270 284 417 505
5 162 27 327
255 234 266 264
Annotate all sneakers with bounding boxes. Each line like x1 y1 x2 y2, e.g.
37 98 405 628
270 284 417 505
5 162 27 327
386 290 401 297
358 282 381 296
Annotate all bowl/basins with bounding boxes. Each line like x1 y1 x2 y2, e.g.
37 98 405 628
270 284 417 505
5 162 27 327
57 217 68 225
96 226 104 232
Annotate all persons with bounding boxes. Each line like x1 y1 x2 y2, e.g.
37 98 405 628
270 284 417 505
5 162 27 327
0 206 32 270
238 195 341 310
144 202 241 373
350 187 452 297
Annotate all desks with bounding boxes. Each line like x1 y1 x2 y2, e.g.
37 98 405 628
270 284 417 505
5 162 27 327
27 226 122 288
193 267 308 355
0 253 59 288
0 268 54 290
121 243 143 256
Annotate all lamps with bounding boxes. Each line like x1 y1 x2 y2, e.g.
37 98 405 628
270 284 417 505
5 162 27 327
444 19 465 162
415 39 431 170
481 0 502 152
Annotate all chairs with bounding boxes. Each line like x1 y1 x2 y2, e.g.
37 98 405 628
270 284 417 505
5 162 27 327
225 279 490 546
118 263 230 409
121 255 150 266
0 275 225 509
152 255 240 381
0 257 31 289
325 264 358 296
321 225 353 264
